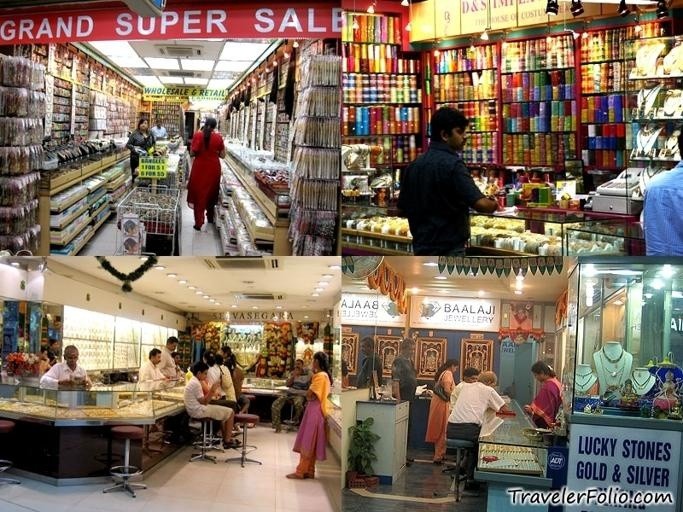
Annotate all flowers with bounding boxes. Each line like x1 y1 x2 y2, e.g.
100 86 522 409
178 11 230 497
4 353 51 376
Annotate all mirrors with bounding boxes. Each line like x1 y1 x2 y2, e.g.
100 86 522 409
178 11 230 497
564 159 583 194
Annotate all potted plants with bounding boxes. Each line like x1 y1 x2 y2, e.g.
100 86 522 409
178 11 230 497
347 415 379 492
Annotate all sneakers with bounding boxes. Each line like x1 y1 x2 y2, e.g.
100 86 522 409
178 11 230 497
276 428 281 433
304 473 314 479
465 481 481 490
294 419 301 426
222 438 241 444
223 442 237 449
193 224 202 230
208 218 213 223
286 473 304 479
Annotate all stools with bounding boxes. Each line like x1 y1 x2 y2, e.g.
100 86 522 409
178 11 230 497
0 419 20 485
432 436 476 505
244 393 257 403
101 426 148 499
282 399 302 429
188 416 223 464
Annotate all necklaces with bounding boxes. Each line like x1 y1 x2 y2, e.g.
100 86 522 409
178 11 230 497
575 375 593 389
575 370 593 379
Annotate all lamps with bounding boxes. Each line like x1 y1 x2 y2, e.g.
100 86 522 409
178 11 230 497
655 0 667 21
546 1 584 18
617 0 630 15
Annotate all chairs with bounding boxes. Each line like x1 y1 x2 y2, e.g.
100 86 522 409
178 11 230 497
224 412 262 469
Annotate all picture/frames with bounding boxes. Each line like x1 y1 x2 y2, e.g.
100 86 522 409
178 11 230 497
342 292 404 328
461 334 494 385
414 337 447 380
408 295 502 333
342 327 361 376
375 335 402 378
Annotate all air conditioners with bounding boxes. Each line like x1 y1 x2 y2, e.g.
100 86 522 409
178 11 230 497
166 72 196 78
201 257 281 273
235 293 284 303
154 44 205 58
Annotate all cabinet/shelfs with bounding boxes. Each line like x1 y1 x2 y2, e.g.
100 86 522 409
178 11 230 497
343 8 420 186
565 259 680 511
425 52 502 200
476 396 553 512
6 38 142 255
2 298 62 359
581 17 674 192
24 306 188 370
338 202 648 255
357 397 409 486
412 1 633 41
0 372 343 485
626 35 681 217
212 30 341 255
498 32 582 189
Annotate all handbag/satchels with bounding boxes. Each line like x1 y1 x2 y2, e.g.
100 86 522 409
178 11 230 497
434 370 450 403
220 367 232 390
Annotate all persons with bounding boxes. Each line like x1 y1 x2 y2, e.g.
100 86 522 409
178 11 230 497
170 351 186 378
212 353 239 415
448 366 480 416
391 338 418 468
643 123 683 257
39 344 93 390
424 302 435 317
121 219 138 236
125 117 156 187
137 347 173 384
522 360 569 432
286 350 334 480
230 363 251 433
219 345 237 380
202 349 227 400
395 106 498 257
186 117 226 232
446 370 510 491
599 345 626 388
342 359 350 390
123 237 139 255
183 360 242 450
148 118 168 145
632 370 652 390
515 302 528 320
156 335 179 380
424 358 459 466
355 336 383 390
270 358 313 434
38 338 58 377
